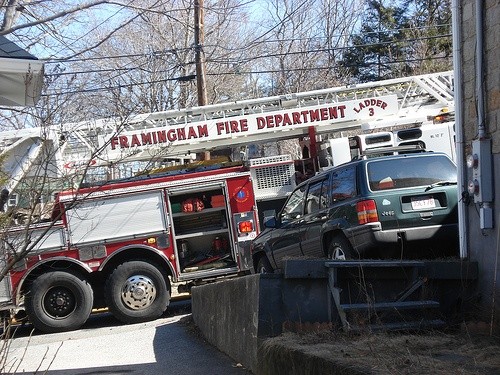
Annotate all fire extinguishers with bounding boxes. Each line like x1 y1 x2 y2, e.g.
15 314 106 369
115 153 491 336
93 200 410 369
213 237 228 252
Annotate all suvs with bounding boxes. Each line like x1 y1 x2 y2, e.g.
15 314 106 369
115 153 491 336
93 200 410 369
251 145 459 276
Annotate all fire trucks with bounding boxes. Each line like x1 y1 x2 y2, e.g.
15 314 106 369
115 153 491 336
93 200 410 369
0 154 297 331
